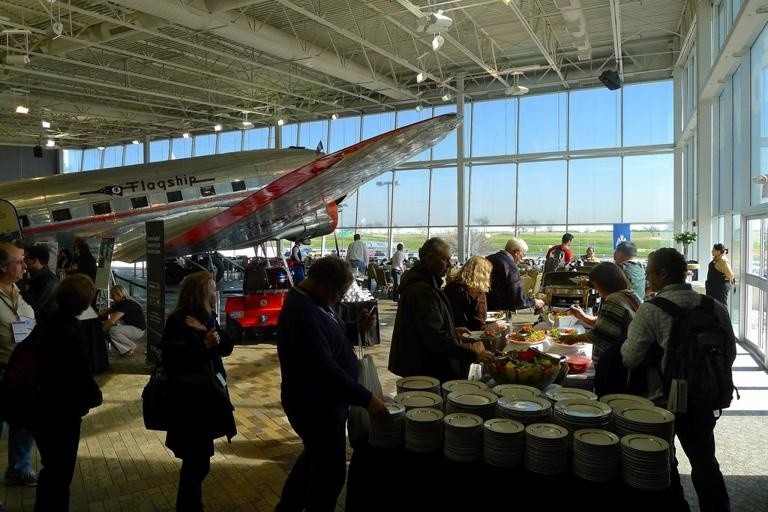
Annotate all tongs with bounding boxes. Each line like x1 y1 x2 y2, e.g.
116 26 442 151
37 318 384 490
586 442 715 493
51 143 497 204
479 350 509 366
559 304 580 330
528 347 560 364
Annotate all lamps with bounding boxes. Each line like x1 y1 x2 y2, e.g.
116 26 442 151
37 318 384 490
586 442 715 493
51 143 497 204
242 115 253 131
274 115 286 128
180 129 191 140
415 95 423 111
41 135 58 150
211 121 223 133
38 117 54 133
327 108 341 124
442 82 451 102
417 55 428 83
504 72 529 96
131 137 142 146
16 104 30 118
432 32 445 52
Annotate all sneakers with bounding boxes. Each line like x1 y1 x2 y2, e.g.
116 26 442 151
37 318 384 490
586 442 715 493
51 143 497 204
4 470 39 486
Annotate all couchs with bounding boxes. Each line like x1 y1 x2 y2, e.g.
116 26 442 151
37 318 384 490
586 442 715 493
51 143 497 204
543 272 596 307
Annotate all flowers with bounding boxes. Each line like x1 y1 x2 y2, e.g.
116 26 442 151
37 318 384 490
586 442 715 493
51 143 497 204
686 270 694 276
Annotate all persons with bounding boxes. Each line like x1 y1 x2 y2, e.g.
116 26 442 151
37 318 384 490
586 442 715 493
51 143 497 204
619 247 734 510
292 234 417 302
159 270 239 512
614 241 646 301
577 247 599 262
276 256 382 512
558 262 642 396
485 237 544 310
705 244 731 305
546 234 574 265
0 240 146 512
443 256 507 368
388 237 496 384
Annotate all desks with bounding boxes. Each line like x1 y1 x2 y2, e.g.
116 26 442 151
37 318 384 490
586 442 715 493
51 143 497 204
691 281 706 296
336 285 380 346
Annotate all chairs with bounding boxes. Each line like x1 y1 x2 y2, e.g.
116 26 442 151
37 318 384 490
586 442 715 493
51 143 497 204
584 262 600 266
518 263 552 307
446 266 462 283
368 262 420 299
577 266 594 272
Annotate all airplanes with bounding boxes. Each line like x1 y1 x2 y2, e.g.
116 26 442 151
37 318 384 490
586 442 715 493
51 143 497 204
0 109 465 283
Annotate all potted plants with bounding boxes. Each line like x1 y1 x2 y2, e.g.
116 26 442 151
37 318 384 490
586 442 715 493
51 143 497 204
672 231 697 264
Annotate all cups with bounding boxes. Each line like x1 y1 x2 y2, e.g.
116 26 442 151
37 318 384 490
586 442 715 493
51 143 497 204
548 313 555 324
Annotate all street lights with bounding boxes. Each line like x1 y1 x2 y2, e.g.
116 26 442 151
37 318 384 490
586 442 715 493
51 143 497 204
376 179 399 257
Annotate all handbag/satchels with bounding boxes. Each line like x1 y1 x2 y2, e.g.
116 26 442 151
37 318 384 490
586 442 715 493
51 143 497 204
142 369 168 431
594 336 645 397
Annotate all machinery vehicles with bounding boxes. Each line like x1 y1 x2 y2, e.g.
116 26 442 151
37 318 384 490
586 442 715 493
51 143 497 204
225 251 308 344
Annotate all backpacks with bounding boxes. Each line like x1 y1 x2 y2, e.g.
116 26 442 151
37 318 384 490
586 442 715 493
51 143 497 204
644 294 739 408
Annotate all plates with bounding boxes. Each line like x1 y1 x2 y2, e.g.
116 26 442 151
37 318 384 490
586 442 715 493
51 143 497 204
599 393 655 405
442 378 489 391
462 330 498 341
486 311 507 322
544 387 598 400
614 404 676 430
443 411 483 467
407 407 443 459
505 333 546 344
447 390 497 410
492 383 542 397
394 390 445 405
525 421 570 480
553 400 613 424
497 396 552 416
483 416 524 475
550 325 586 346
572 428 618 488
621 433 673 493
395 376 441 390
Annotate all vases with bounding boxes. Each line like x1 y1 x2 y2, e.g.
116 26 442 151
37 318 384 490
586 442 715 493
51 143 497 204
685 275 692 284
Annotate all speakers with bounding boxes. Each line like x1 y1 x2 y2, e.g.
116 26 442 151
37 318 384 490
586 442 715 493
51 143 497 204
33 145 43 157
599 70 621 90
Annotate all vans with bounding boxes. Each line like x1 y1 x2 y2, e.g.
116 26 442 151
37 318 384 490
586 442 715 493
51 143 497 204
283 249 386 260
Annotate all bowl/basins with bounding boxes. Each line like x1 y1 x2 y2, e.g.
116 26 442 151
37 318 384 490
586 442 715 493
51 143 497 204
540 312 580 328
567 355 591 373
569 278 590 285
485 366 561 388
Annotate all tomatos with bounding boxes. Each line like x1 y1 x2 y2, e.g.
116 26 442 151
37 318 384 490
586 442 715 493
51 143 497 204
518 350 535 363
